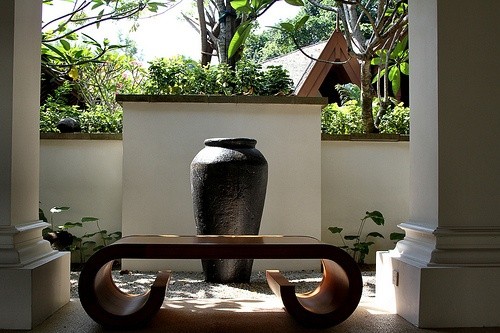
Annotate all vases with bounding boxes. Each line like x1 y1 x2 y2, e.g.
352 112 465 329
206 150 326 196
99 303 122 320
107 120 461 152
189 136 271 283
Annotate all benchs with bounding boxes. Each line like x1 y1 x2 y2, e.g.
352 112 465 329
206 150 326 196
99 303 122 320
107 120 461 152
77 234 365 331
